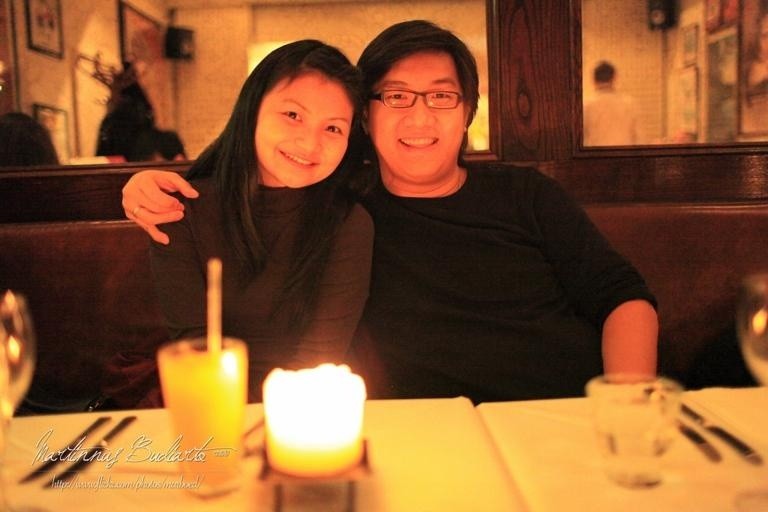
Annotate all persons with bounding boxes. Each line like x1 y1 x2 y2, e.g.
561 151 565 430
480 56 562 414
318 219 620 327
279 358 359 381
147 39 390 403
153 131 187 162
583 61 638 147
121 20 659 405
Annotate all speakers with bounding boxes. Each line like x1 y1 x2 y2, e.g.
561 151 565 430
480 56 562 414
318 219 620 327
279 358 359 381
648 0 681 29
163 25 193 61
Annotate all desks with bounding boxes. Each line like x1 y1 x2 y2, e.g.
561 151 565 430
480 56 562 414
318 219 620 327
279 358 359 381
0 384 767 511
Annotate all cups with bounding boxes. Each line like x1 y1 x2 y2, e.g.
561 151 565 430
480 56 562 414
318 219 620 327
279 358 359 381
157 332 253 500
583 373 688 487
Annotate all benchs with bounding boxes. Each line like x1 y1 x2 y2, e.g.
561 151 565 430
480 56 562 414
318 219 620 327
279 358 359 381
0 193 765 409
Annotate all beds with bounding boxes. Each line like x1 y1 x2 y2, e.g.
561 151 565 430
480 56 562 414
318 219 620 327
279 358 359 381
0 384 767 511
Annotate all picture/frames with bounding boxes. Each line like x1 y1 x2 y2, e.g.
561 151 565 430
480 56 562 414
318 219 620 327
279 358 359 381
34 102 72 161
25 1 65 57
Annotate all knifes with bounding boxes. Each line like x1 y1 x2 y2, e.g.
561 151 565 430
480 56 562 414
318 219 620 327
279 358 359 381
674 393 765 469
19 413 138 490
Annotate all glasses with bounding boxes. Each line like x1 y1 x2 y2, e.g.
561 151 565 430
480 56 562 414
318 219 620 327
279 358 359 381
371 90 464 109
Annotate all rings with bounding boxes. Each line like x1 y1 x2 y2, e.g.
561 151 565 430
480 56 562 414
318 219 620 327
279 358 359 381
132 206 141 216
